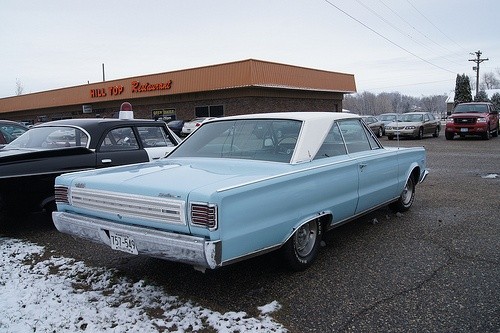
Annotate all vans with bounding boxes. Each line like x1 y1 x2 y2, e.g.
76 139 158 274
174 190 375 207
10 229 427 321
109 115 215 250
379 113 402 133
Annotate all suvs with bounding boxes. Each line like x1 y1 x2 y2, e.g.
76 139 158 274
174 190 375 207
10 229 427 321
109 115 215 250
444 102 500 140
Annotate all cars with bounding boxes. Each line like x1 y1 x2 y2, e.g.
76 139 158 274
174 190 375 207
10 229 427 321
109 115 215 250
54 111 426 268
0 119 184 218
386 113 440 140
0 121 87 147
254 121 300 140
166 119 190 137
334 116 385 142
182 117 236 135
